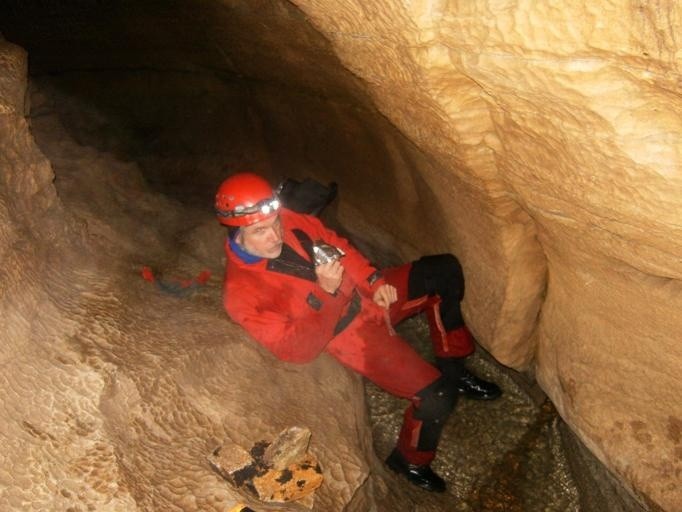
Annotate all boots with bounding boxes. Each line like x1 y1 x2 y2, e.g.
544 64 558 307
434 361 503 400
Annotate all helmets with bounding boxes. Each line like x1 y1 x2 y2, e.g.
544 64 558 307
214 170 280 227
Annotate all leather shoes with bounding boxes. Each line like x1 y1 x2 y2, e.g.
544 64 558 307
384 447 447 492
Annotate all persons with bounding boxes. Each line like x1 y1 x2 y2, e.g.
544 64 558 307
214 172 503 493
